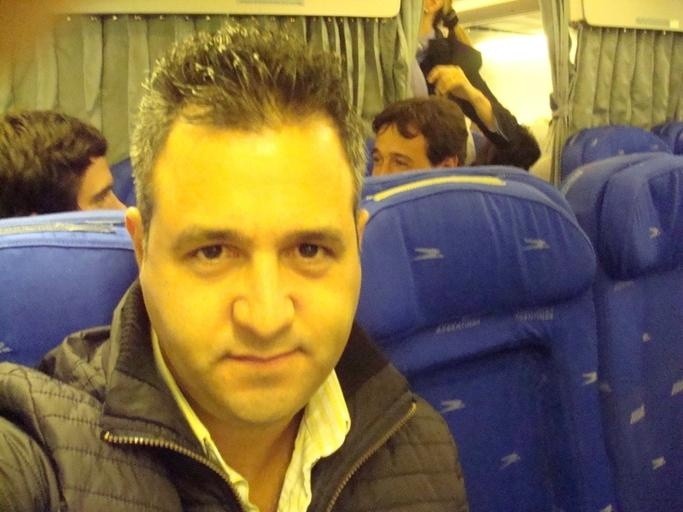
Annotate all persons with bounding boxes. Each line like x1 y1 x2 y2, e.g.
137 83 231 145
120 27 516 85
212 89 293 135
424 61 541 173
1 19 473 512
366 62 469 181
1 106 129 219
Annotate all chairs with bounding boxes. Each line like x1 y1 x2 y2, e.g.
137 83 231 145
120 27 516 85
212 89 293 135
0 119 683 512
